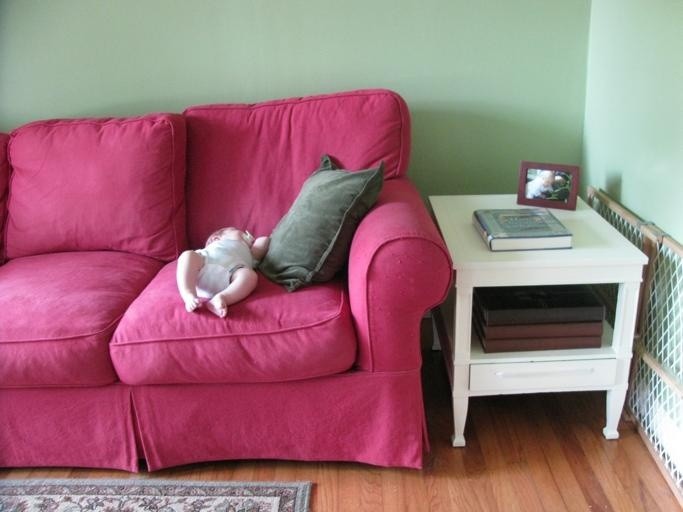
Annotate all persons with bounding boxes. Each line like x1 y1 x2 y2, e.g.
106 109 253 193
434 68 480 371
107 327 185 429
523 169 555 199
174 225 268 317
545 172 571 201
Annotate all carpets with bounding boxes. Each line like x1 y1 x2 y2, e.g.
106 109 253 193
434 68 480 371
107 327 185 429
0 478 313 510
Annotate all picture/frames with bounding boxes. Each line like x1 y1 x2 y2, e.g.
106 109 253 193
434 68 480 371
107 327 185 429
518 159 580 212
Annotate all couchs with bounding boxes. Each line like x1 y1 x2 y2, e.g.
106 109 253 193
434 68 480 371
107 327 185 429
3 95 453 470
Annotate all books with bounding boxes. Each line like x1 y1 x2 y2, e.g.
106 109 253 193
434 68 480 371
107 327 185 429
473 206 573 251
472 318 601 353
474 311 603 340
473 283 604 325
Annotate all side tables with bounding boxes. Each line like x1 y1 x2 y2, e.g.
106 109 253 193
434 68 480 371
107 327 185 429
428 192 650 447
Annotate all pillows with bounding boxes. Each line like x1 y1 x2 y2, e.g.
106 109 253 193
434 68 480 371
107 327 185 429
258 158 387 295
